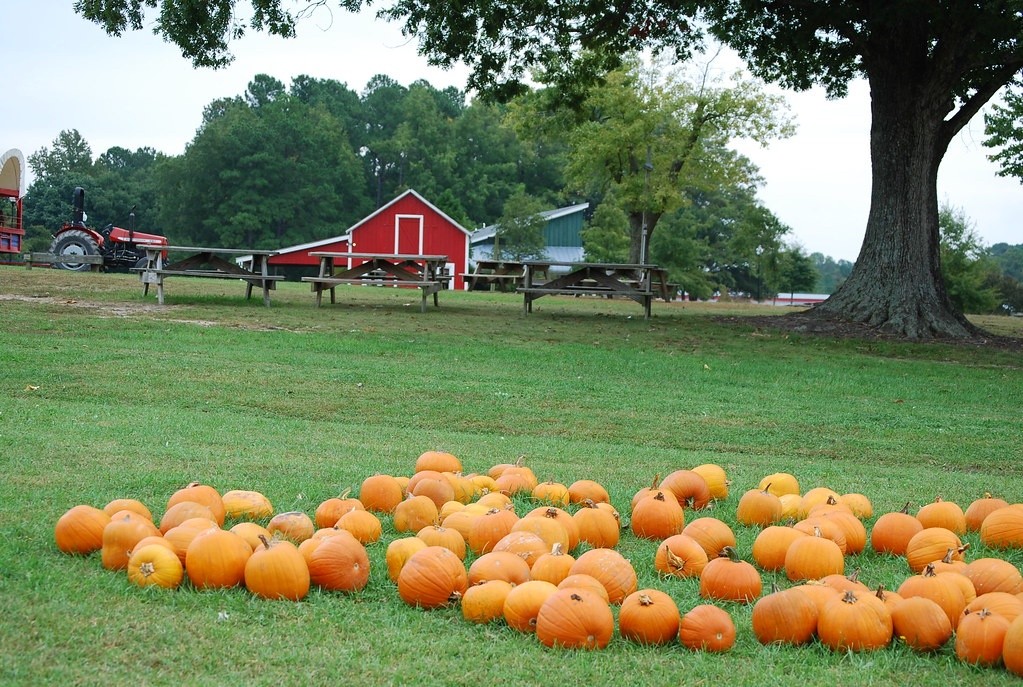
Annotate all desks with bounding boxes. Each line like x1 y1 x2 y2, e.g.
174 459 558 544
135 244 280 308
519 261 668 320
308 252 448 313
467 260 552 294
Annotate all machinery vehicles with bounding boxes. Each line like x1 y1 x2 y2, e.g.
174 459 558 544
0 146 168 277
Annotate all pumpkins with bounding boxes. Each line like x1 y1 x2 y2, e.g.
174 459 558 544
54 450 1023 679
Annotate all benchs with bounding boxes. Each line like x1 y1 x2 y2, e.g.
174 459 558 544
301 276 453 313
458 273 523 294
129 268 285 308
515 281 681 320
